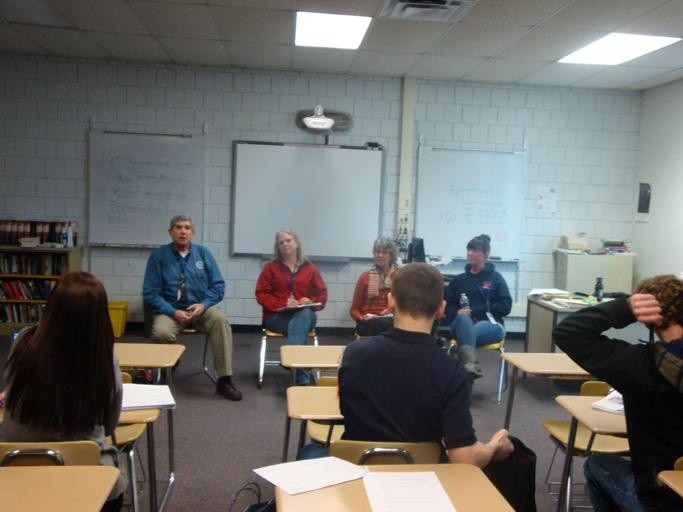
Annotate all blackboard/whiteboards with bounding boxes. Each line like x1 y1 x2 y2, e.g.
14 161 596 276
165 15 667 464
414 145 527 260
230 140 385 260
87 130 205 248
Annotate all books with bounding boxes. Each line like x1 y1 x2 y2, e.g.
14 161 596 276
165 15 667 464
0 221 71 323
591 390 625 415
121 383 177 411
555 234 625 255
528 288 590 309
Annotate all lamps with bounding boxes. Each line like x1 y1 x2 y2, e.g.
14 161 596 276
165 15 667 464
295 105 353 145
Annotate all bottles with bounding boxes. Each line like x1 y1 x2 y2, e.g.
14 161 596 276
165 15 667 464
594 276 603 302
60 225 74 247
458 292 471 317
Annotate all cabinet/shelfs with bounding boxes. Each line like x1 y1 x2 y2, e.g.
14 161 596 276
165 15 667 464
0 245 82 336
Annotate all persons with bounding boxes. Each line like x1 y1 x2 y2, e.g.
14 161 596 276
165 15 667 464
349 235 400 337
551 273 683 512
444 233 513 405
142 214 242 401
296 261 514 470
255 227 327 386
0 271 129 512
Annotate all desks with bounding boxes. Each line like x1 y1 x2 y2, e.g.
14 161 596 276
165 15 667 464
0 343 186 512
281 384 345 463
499 353 683 512
274 460 518 512
554 249 634 295
523 298 617 378
280 345 345 386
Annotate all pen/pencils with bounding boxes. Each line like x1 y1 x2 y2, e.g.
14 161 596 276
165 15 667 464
289 291 294 299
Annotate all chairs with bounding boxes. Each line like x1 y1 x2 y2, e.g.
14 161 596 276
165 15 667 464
328 439 443 467
257 319 320 389
156 329 216 383
307 373 346 444
447 339 505 405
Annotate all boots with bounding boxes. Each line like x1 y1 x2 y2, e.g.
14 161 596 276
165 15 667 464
458 344 483 378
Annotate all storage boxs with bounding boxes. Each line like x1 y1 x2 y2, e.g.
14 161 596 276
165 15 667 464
107 301 128 338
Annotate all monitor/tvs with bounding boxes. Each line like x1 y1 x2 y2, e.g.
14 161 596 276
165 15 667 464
412 237 425 263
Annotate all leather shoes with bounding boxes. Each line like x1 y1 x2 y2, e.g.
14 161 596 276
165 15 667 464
216 379 241 401
